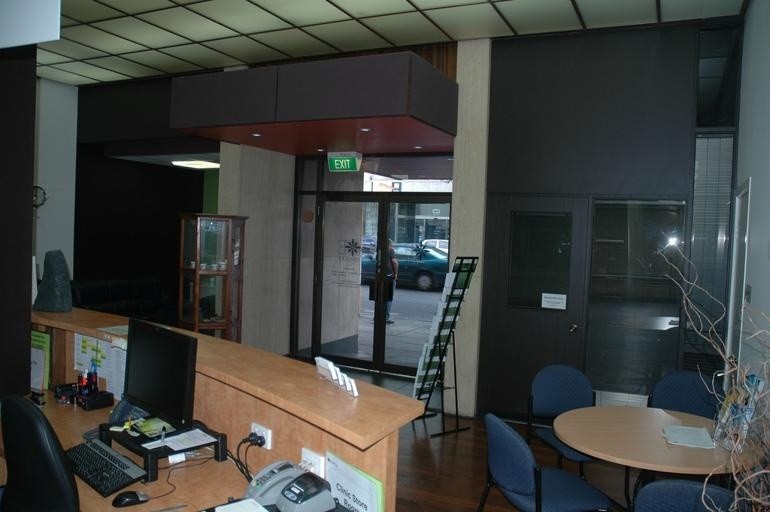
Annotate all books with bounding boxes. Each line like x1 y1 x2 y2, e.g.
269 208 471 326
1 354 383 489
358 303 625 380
664 425 716 450
714 373 765 455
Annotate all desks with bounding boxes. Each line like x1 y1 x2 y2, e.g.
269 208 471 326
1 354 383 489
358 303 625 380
553 405 764 512
0 382 253 512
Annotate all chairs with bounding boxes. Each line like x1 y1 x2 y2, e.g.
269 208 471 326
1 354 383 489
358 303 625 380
525 364 596 479
0 394 81 512
633 469 755 512
632 371 727 504
478 413 614 512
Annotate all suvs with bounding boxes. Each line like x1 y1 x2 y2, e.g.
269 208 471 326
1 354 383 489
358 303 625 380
422 238 450 253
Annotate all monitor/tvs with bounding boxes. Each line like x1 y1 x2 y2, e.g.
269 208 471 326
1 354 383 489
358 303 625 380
123 317 197 430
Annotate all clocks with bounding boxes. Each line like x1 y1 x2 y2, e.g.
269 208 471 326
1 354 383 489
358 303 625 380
33 186 46 208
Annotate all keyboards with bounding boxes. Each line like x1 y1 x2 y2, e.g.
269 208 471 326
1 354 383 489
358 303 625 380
65 438 147 498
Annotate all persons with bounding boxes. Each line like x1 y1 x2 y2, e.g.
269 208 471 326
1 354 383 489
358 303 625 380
385 239 399 323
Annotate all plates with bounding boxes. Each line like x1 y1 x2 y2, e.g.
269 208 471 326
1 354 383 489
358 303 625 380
210 316 228 323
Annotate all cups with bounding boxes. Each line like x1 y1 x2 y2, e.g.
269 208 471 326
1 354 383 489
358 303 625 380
78 375 89 397
211 264 218 271
218 262 228 270
87 372 99 395
190 261 196 269
199 264 207 270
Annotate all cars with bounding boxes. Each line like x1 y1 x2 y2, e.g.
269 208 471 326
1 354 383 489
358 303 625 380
362 245 448 290
363 236 378 248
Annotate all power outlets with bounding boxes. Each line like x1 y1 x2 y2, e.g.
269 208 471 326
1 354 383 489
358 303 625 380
250 423 271 451
301 447 324 479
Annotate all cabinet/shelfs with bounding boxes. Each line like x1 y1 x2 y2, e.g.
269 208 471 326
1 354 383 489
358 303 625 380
178 212 249 344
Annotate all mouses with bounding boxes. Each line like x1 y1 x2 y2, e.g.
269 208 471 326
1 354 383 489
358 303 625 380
112 490 151 507
